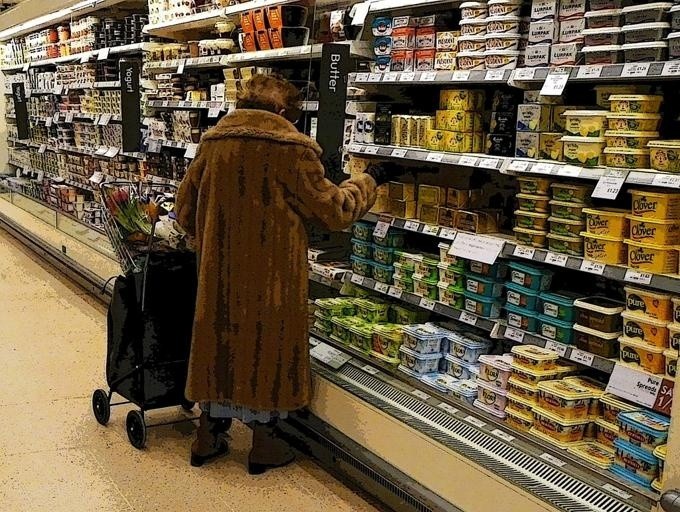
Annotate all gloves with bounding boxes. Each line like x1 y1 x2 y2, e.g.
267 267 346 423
363 161 405 185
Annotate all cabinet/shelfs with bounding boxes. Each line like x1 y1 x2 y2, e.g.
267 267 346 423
1 0 143 234
145 1 680 502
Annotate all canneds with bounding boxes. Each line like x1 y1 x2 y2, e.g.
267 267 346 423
355 113 375 145
60 15 99 56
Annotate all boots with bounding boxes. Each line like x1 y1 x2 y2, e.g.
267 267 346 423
248 421 296 475
190 412 228 466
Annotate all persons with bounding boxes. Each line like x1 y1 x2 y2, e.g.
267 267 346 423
174 74 398 474
